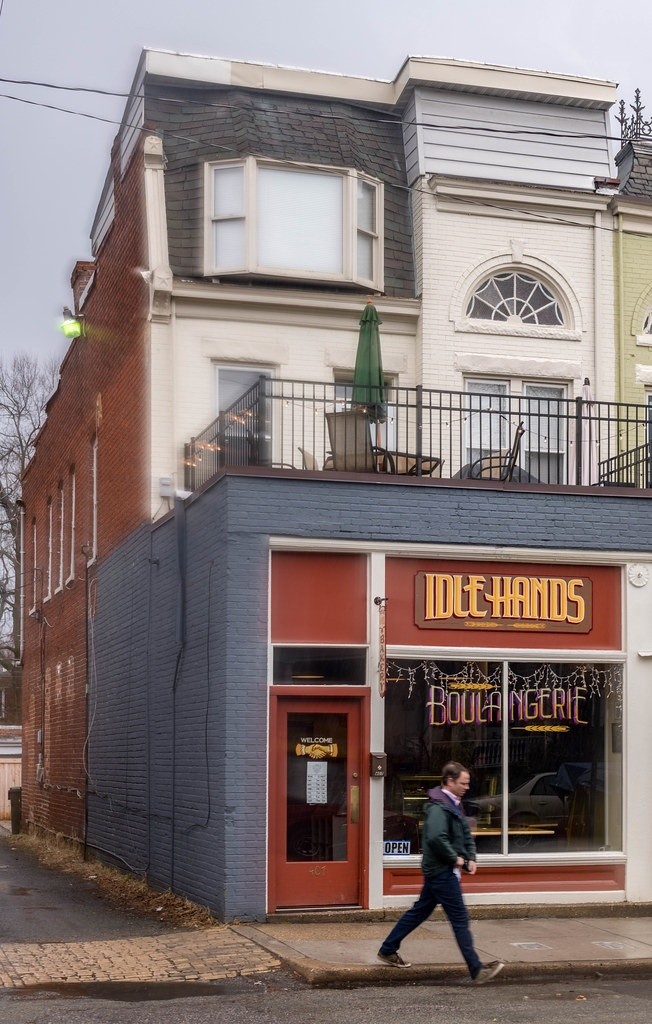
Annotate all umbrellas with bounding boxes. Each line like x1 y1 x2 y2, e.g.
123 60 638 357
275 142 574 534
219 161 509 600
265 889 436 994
349 300 387 447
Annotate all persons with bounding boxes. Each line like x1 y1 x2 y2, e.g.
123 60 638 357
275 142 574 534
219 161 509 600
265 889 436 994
378 762 506 984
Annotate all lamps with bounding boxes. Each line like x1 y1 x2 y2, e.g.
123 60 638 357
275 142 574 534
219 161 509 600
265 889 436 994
61 306 86 339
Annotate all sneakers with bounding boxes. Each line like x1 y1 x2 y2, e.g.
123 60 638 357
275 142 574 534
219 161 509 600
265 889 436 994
376 951 411 968
472 960 505 985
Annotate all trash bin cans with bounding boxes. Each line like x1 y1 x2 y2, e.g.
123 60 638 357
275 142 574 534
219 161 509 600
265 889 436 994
7 786 21 835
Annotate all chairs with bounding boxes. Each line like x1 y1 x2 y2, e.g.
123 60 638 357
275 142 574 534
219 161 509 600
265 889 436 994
325 410 395 475
467 421 526 482
383 815 420 855
299 446 319 470
246 428 299 469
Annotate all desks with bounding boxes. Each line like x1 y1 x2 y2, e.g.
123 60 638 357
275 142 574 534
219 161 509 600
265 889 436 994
323 450 446 478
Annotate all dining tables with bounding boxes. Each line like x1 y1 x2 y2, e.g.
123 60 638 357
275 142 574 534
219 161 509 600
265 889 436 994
471 827 554 853
